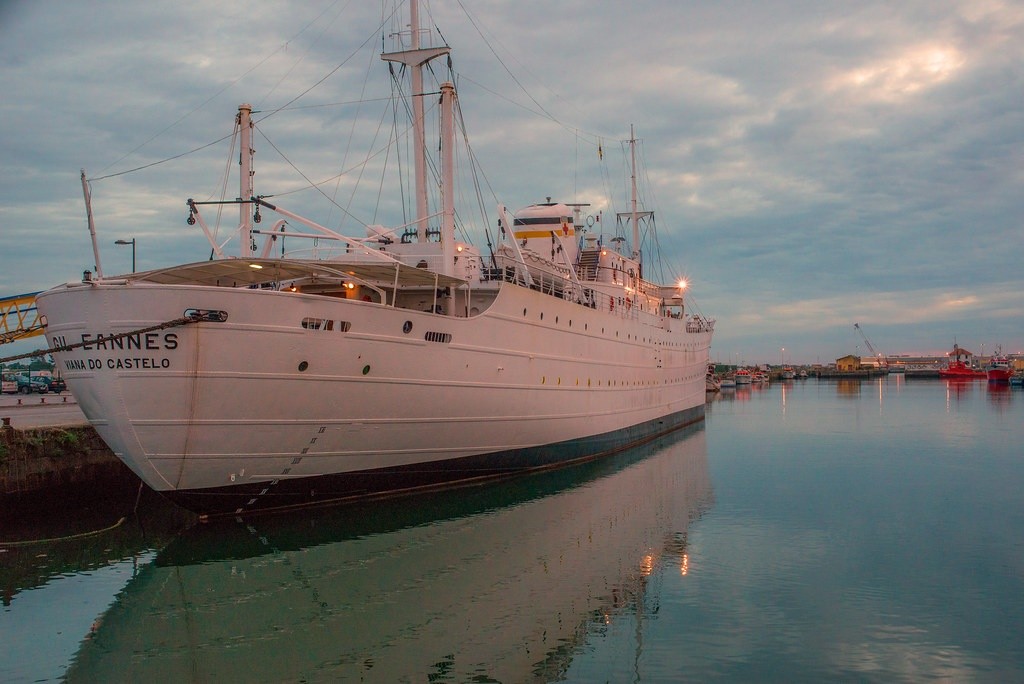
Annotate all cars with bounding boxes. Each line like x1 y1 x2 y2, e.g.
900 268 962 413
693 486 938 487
17 374 49 395
30 374 66 393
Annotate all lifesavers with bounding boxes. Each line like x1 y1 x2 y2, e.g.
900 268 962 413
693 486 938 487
609 296 615 312
625 296 633 311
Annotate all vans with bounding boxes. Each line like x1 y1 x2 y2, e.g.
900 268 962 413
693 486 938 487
2 371 18 395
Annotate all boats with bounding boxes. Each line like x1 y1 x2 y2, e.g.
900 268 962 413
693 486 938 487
720 372 736 387
939 349 987 377
782 368 794 379
799 370 809 378
1007 376 1024 384
706 364 721 392
736 368 753 384
986 352 1013 380
32 1 717 519
753 371 770 383
